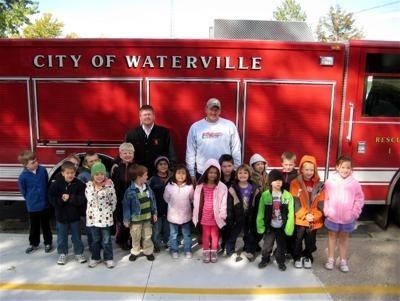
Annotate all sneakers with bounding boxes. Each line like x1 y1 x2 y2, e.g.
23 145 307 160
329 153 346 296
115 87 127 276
258 257 270 268
74 254 87 263
278 261 286 271
129 254 136 261
57 253 68 265
339 258 349 272
88 259 103 267
104 260 115 268
325 256 336 269
45 245 51 253
147 254 154 260
295 257 303 268
303 256 312 268
172 252 178 258
25 245 39 254
186 252 192 259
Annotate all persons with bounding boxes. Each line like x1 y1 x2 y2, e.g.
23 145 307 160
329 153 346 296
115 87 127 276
217 153 236 253
84 162 117 268
148 155 173 253
162 165 195 259
122 164 158 261
77 152 109 252
273 152 299 263
192 158 228 263
110 142 136 250
290 155 325 269
185 97 242 243
125 104 171 179
222 163 261 262
17 150 53 254
47 154 81 206
249 153 269 253
256 169 295 271
323 156 365 272
53 160 87 265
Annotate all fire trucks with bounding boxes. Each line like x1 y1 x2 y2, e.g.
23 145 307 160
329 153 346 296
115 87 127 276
0 36 400 230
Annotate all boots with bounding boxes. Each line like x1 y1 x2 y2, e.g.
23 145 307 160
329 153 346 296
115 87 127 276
203 250 211 263
211 250 217 263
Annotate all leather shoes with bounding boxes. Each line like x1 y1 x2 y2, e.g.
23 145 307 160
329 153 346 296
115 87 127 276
120 241 130 250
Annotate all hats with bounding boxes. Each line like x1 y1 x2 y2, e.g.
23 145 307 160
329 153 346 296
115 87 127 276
153 153 169 168
207 97 220 109
91 162 106 178
